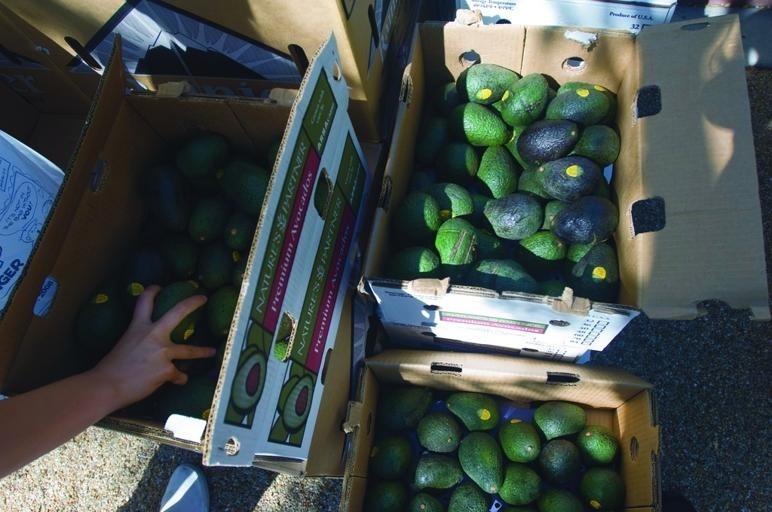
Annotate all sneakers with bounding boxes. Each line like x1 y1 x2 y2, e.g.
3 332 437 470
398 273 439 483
160 464 209 512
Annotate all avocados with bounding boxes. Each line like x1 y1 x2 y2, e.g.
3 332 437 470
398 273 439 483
54 108 281 411
384 62 621 303
363 387 624 512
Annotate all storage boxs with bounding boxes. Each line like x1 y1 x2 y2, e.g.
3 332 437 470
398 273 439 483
358 12 771 357
181 1 397 128
336 349 664 512
0 33 371 478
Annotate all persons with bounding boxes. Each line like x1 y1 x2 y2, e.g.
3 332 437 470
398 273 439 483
0 285 217 512
